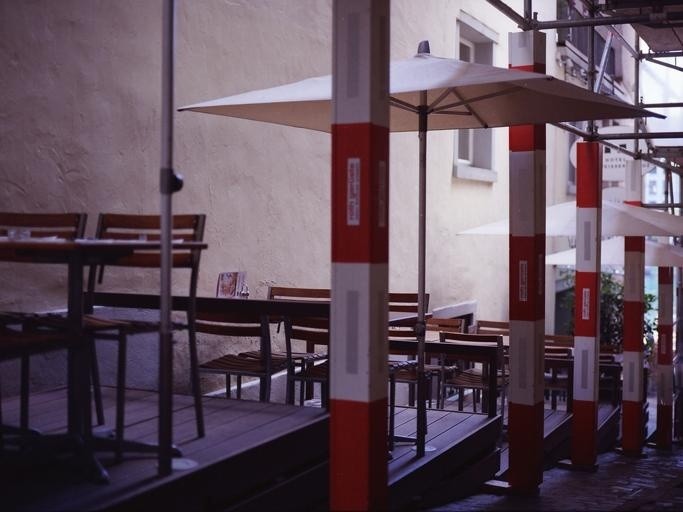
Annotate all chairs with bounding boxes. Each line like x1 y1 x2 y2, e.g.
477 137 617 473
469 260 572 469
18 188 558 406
204 285 621 452
0 209 108 435
91 206 208 443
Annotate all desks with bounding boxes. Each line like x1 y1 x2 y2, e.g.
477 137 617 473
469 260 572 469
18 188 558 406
1 236 207 482
